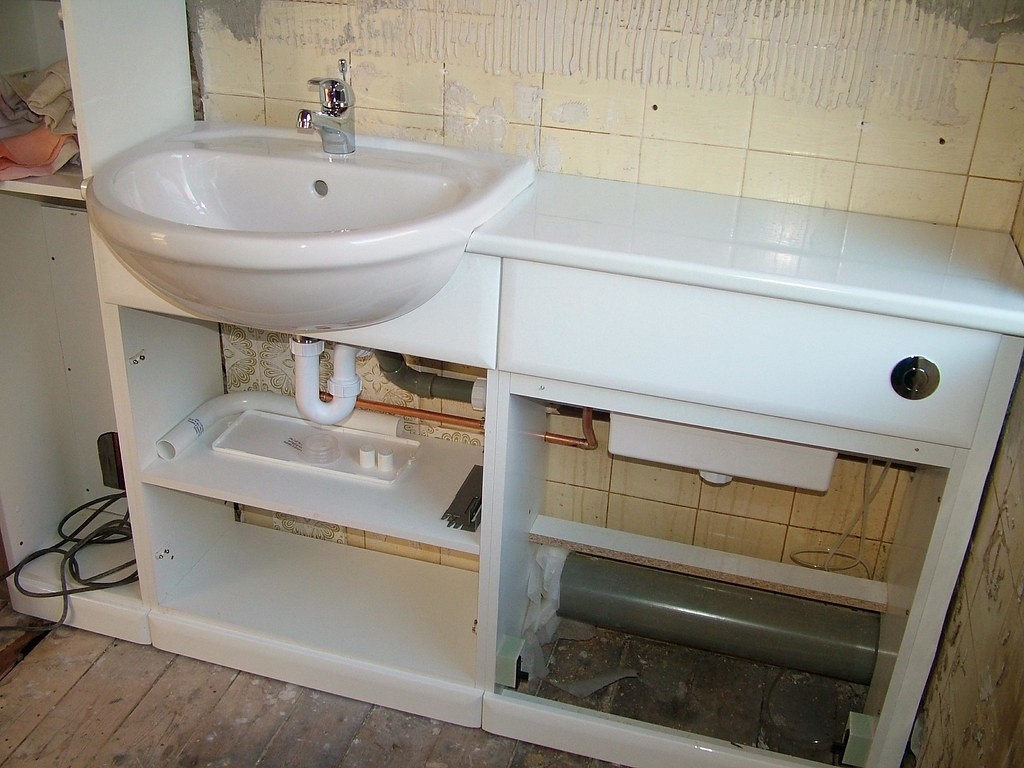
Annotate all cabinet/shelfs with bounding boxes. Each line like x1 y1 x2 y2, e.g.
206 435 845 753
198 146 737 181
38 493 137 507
0 0 150 652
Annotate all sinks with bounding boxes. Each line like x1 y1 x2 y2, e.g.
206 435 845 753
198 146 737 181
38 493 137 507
84 110 538 339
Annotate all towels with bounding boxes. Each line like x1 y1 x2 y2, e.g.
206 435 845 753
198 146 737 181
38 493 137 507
0 55 85 188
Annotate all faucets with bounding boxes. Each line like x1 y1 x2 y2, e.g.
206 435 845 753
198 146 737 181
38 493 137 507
291 56 361 157
